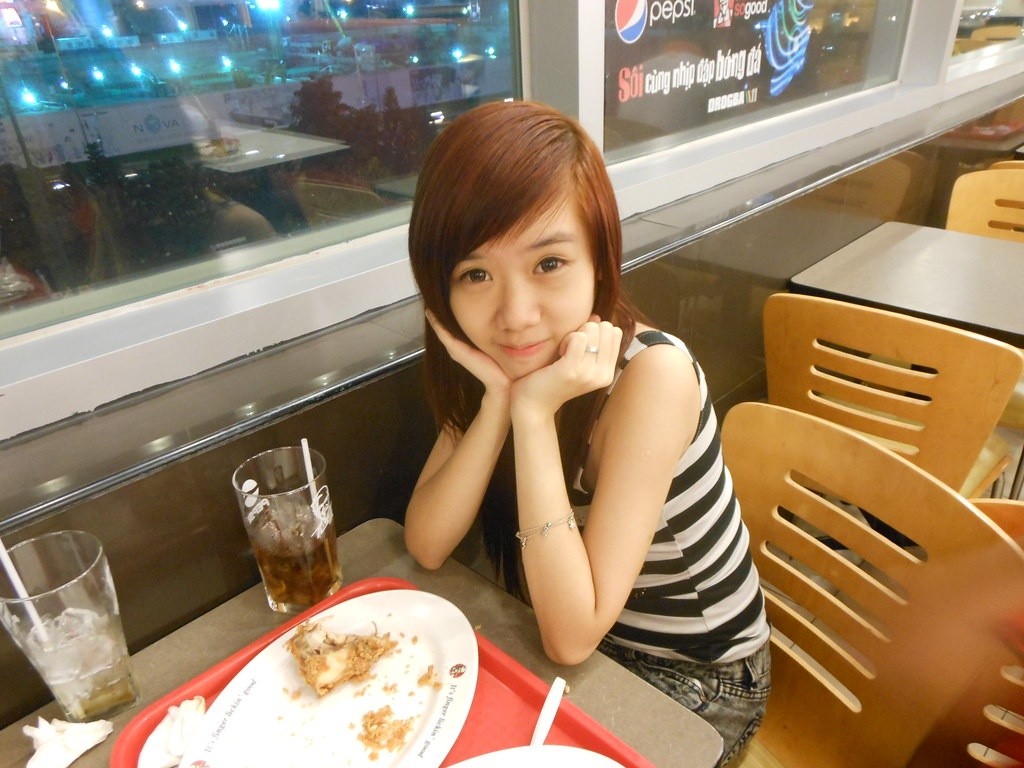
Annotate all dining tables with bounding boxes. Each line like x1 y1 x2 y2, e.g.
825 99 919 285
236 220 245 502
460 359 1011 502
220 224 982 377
925 120 1024 151
664 202 888 294
197 120 350 238
0 517 726 768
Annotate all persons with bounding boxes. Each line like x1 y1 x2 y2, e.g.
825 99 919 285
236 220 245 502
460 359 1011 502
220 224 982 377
64 96 277 283
403 100 772 768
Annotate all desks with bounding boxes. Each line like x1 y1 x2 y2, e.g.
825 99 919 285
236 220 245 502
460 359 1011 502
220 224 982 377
791 217 1024 350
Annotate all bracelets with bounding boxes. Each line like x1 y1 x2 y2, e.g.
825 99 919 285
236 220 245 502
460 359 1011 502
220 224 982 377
515 510 574 549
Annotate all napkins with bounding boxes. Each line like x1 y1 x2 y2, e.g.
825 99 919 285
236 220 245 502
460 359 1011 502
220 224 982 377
19 717 112 768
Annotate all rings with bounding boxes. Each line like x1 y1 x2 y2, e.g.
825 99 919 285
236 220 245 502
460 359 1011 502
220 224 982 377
586 347 598 353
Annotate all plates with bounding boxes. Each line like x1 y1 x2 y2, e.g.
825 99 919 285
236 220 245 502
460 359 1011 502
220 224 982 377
0 281 34 303
199 145 242 163
445 745 626 768
177 590 479 768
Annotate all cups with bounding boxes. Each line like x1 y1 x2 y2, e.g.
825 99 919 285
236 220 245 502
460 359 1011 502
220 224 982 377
232 447 342 615
0 531 140 723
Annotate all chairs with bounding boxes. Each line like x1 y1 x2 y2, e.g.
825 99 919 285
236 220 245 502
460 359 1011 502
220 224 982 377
911 498 1024 768
995 97 1024 122
765 290 1018 502
716 403 1024 768
794 156 912 220
945 167 1024 242
